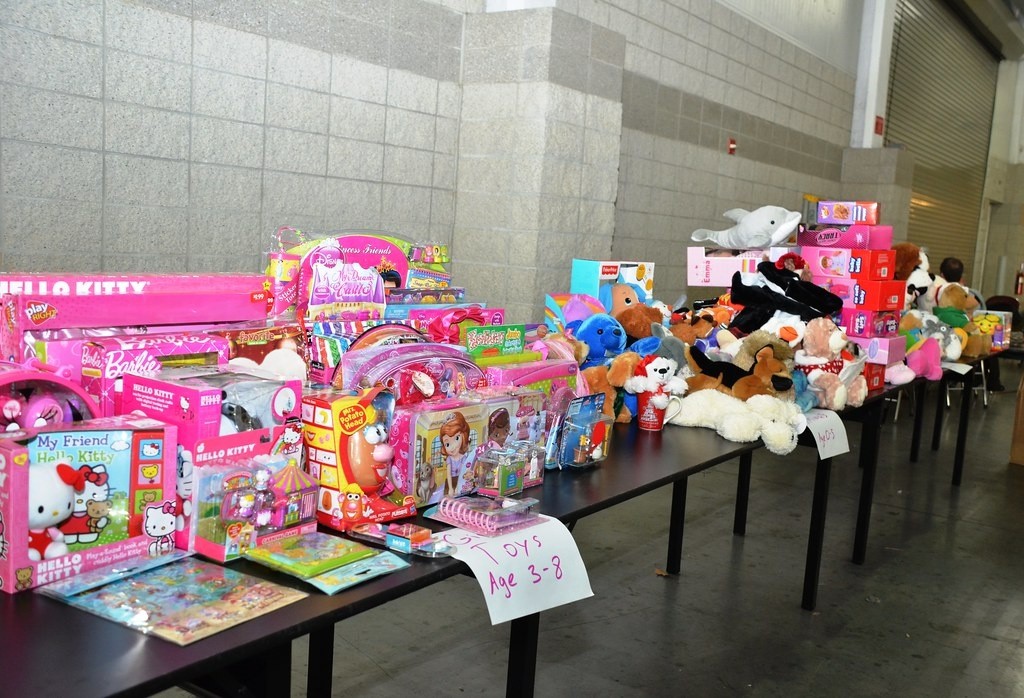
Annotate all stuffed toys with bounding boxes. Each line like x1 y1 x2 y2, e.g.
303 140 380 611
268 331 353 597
546 243 992 458
690 205 802 251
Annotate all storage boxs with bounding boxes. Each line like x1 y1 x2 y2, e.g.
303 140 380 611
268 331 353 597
0 226 656 597
689 199 1011 392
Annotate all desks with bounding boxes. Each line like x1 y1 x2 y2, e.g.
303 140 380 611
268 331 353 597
0 352 975 698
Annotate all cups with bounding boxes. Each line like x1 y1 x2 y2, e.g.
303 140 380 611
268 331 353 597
636 386 681 432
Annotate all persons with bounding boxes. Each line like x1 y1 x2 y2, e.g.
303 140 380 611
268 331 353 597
939 256 1006 391
